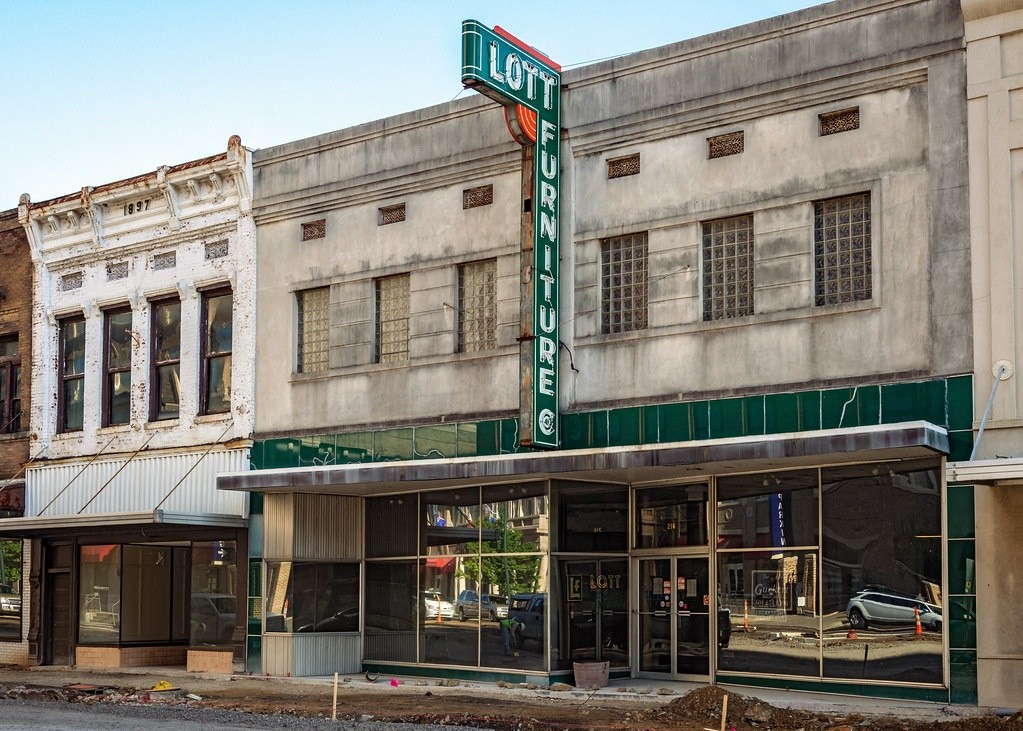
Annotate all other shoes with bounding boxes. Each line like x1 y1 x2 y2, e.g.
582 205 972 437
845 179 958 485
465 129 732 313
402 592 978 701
505 652 512 656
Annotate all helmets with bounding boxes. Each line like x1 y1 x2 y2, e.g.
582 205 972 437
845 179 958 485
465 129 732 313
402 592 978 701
521 623 525 631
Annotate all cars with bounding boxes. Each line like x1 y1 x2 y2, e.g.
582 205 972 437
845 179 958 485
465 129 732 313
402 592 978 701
844 584 942 633
507 592 544 652
190 591 237 646
0 595 21 617
424 587 453 621
315 606 359 632
455 589 509 622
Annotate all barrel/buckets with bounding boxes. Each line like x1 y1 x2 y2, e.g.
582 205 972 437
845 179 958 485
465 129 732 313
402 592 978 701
573 661 610 689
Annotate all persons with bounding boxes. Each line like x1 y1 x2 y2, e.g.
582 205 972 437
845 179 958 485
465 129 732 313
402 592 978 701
500 619 525 656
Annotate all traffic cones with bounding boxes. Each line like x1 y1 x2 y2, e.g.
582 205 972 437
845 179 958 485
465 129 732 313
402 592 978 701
914 606 923 636
